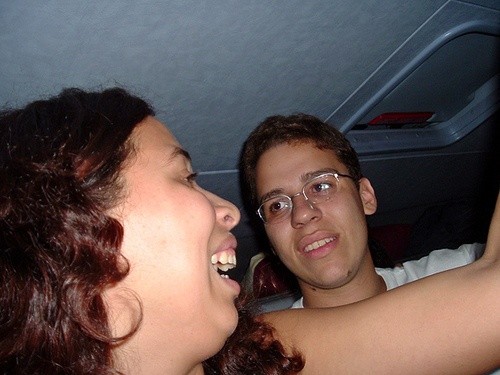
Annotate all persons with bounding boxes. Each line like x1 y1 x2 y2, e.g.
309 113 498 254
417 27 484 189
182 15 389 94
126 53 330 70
238 113 500 374
0 86 500 375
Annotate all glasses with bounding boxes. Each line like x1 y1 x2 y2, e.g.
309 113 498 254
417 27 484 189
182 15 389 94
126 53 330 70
256 173 354 224
